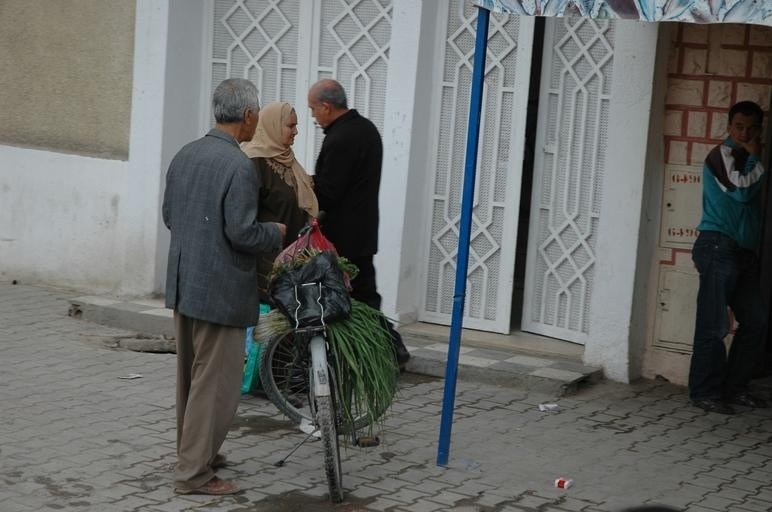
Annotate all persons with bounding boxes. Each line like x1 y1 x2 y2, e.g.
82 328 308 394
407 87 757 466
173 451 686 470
309 79 409 364
688 101 769 414
239 100 319 409
162 77 287 495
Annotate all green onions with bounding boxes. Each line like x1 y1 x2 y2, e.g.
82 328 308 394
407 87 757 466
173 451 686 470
253 295 400 452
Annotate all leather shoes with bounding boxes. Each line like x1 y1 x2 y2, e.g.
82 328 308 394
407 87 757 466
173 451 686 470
735 394 766 409
174 475 240 495
212 454 227 469
693 398 736 415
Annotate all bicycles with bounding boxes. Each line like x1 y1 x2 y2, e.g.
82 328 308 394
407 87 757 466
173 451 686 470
258 209 397 503
